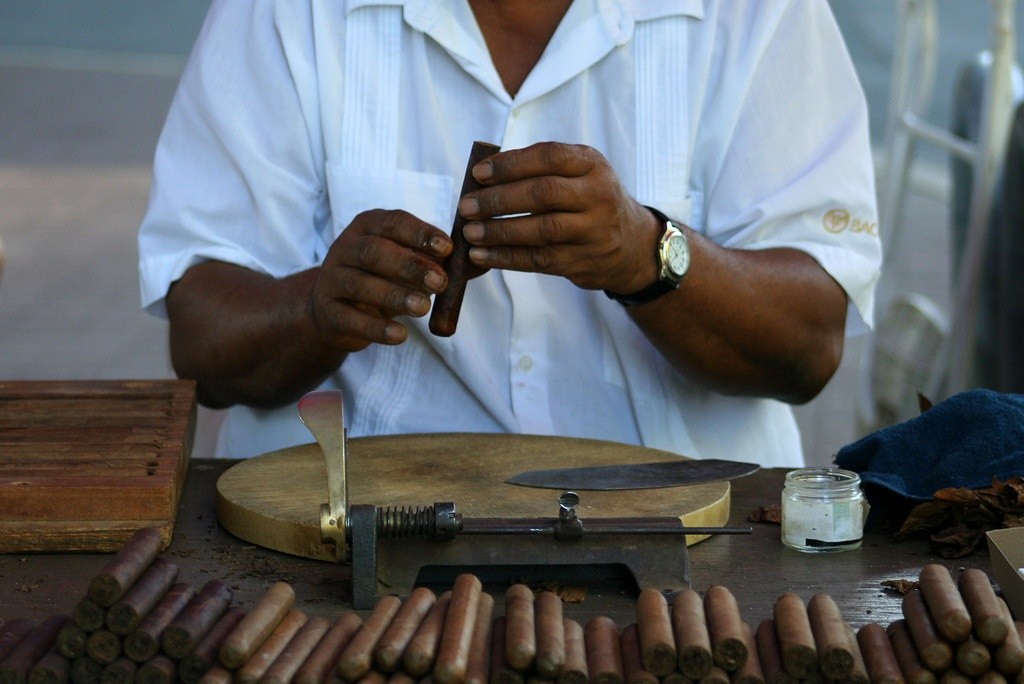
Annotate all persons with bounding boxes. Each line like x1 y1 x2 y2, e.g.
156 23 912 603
137 0 884 469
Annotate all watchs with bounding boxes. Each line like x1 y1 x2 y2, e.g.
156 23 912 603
602 205 691 306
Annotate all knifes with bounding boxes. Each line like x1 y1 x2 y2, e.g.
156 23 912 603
504 459 760 492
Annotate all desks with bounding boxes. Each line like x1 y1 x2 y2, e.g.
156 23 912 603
0 459 1024 684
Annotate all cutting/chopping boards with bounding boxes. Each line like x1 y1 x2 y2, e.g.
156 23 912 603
215 431 730 568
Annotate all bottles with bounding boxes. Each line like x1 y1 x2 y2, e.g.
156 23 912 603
781 470 866 552
